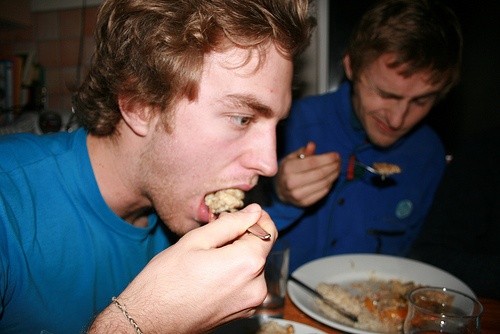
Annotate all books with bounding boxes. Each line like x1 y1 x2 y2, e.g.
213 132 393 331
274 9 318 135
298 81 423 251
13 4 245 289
0 48 39 123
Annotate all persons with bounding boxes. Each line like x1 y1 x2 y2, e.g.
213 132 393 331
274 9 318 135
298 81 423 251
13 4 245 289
238 0 462 334
0 0 318 334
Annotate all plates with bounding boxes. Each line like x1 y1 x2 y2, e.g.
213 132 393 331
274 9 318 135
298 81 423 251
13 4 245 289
286 254 475 334
262 318 326 334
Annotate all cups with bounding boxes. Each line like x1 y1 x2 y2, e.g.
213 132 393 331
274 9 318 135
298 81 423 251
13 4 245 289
254 241 290 319
404 286 483 334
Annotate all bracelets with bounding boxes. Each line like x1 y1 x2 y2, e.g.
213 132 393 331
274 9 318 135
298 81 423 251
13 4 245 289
112 296 143 334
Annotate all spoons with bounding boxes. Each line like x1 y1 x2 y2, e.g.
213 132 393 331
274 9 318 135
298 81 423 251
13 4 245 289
299 152 398 174
